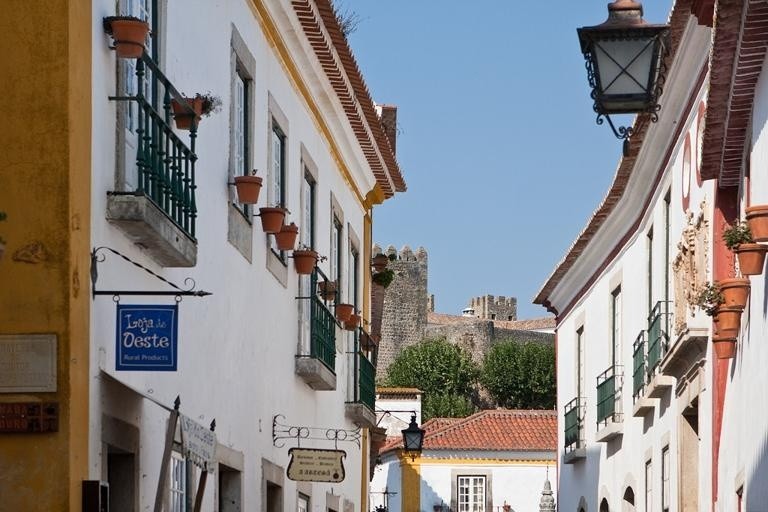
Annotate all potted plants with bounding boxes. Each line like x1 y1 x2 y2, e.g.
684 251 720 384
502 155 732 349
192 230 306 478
698 280 745 340
258 205 284 234
275 224 298 249
318 280 383 345
232 169 263 204
722 224 768 275
171 92 203 132
293 248 317 274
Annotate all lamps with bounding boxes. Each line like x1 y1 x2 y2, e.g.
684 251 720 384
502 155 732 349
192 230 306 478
575 0 673 155
372 410 426 462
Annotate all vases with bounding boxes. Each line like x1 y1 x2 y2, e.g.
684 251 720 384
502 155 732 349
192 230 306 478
745 205 768 242
712 335 737 359
717 278 751 309
